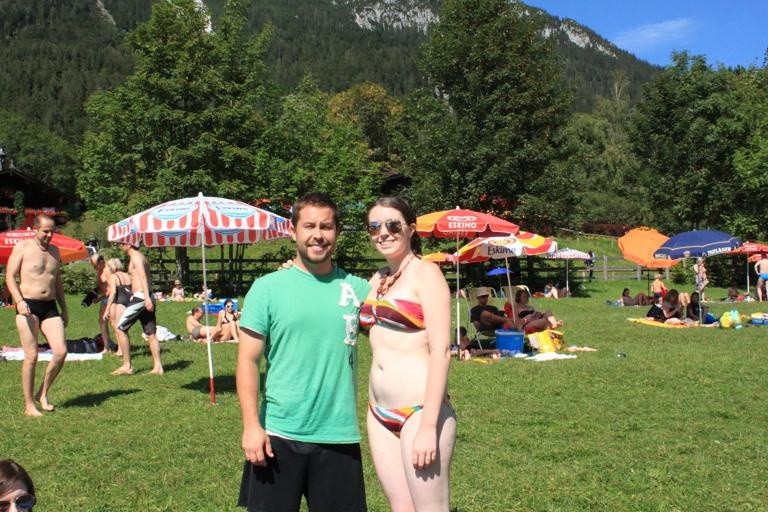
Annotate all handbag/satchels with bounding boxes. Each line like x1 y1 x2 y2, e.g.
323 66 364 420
66 333 117 353
647 304 662 318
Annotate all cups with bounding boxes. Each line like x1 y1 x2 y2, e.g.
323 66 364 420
678 306 684 315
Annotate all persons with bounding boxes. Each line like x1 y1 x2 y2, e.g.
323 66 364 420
281 196 458 512
236 195 371 512
91 241 165 377
5 213 69 417
186 284 241 343
171 279 184 300
0 459 36 512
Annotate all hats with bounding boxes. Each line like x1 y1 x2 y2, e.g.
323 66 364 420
174 280 182 285
476 287 489 297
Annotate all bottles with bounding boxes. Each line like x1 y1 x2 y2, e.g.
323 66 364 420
615 351 628 359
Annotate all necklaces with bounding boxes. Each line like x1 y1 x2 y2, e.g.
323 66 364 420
376 253 414 295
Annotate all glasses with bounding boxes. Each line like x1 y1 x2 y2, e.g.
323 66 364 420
226 304 234 308
370 220 406 234
1 494 36 512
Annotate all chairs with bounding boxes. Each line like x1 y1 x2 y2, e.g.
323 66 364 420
463 287 503 352
500 285 536 338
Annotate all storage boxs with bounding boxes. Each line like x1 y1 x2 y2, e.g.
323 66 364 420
495 329 526 353
